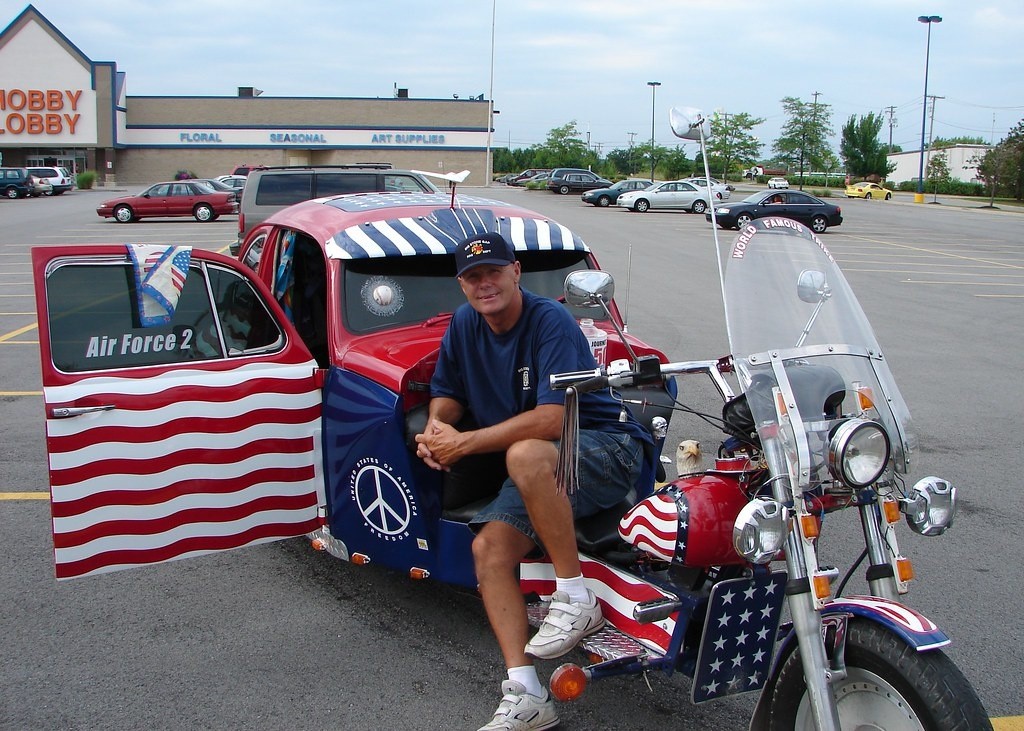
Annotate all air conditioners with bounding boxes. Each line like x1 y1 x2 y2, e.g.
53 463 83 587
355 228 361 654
237 86 254 96
398 89 408 97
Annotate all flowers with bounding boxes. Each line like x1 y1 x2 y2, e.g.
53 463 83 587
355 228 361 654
175 169 197 181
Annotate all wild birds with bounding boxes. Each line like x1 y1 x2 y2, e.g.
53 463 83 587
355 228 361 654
676 439 705 480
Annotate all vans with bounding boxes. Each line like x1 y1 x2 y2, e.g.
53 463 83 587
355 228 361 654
237 163 439 252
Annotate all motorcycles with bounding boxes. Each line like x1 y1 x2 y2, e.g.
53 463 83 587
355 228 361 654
511 219 993 731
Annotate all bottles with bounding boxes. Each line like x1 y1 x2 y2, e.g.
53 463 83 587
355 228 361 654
579 318 607 371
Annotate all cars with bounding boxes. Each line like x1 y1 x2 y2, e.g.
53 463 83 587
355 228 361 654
95 178 246 225
767 177 789 188
497 167 613 194
0 163 73 200
705 188 844 234
34 182 672 586
580 178 656 208
846 182 893 202
679 176 732 199
617 180 719 214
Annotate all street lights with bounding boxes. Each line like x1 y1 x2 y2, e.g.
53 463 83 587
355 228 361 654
647 80 660 180
916 15 941 203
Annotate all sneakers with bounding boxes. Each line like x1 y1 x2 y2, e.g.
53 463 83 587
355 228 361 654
476 679 560 731
524 588 606 661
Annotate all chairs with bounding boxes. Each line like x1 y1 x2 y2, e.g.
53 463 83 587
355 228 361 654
782 197 786 204
404 388 675 528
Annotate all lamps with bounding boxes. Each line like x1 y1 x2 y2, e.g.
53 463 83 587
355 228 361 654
107 162 113 169
453 94 458 99
469 96 475 100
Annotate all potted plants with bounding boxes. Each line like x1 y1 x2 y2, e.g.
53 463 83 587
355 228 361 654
75 170 97 189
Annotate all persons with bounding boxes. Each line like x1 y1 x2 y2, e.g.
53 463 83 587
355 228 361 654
415 233 646 731
773 195 782 203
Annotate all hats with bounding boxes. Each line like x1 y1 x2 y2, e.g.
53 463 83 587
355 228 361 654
454 232 515 279
